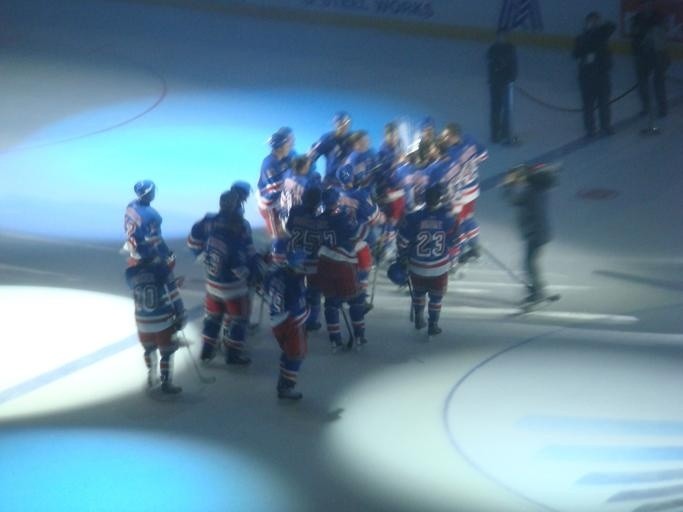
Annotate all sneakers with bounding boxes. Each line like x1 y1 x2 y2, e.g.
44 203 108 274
356 337 367 344
279 387 302 400
415 322 441 334
202 352 214 361
227 356 250 365
161 383 182 395
307 322 321 330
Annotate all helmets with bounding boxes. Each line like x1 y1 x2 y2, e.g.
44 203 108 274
302 187 338 208
387 262 406 284
134 180 154 199
220 182 250 208
136 242 159 260
336 164 353 183
270 127 292 149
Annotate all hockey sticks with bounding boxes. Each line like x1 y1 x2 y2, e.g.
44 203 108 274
339 304 352 352
403 273 415 324
247 288 265 333
475 242 560 303
367 242 380 310
180 324 217 383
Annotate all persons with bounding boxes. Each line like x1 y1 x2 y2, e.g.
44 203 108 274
227 181 254 216
626 1 670 121
183 188 263 368
487 27 525 147
124 178 177 272
124 238 188 396
567 9 618 139
497 159 560 309
256 111 488 399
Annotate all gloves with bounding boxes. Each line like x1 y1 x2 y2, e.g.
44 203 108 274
174 309 187 330
